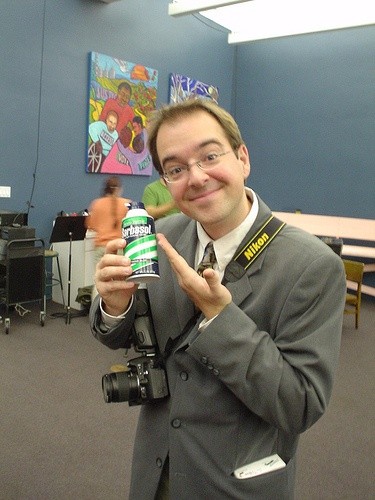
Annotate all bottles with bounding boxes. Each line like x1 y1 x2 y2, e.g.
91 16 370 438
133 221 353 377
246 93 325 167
121 201 160 282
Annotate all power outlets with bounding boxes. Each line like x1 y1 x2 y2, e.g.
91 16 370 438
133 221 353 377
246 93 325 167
0 186 11 197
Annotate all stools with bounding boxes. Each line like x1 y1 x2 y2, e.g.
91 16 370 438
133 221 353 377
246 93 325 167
45 250 67 310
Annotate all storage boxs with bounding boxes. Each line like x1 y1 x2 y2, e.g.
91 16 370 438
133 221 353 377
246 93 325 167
232 454 288 479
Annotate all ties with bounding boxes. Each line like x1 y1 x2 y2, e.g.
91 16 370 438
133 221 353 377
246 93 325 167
195 243 217 278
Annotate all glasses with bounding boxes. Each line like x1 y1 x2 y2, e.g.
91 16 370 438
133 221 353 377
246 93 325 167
160 149 233 183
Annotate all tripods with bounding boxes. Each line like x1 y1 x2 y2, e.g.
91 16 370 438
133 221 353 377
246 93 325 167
49 216 87 325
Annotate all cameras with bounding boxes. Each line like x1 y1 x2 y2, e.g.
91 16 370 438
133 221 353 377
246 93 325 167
102 283 170 407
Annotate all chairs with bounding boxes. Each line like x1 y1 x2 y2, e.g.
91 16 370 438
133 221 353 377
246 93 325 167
343 260 365 330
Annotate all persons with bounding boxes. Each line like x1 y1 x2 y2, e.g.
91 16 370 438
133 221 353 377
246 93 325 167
88 98 347 500
140 176 182 222
85 176 133 287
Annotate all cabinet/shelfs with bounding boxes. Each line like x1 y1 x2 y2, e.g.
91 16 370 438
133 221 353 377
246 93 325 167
52 221 96 311
